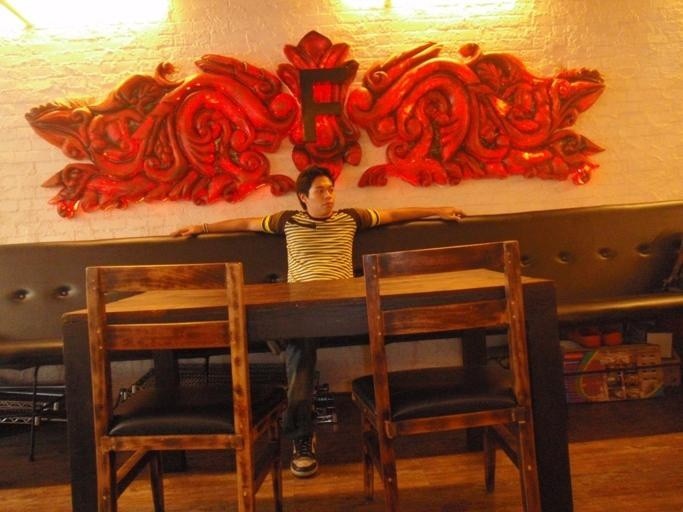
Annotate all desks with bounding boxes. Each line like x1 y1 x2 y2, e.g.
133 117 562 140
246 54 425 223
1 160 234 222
61 268 574 511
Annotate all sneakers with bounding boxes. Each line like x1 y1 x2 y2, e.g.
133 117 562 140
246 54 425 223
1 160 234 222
291 432 318 477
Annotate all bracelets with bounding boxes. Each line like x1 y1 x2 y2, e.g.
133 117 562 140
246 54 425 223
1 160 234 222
202 223 208 233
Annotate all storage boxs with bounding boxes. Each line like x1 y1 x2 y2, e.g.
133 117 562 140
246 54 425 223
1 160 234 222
560 341 665 404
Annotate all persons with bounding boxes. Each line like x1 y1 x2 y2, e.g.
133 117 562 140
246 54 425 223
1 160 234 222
168 165 467 479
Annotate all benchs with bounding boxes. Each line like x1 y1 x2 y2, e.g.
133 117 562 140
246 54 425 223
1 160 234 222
0 200 683 370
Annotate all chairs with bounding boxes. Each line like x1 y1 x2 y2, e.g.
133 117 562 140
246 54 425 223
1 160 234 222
351 240 542 512
86 262 289 512
